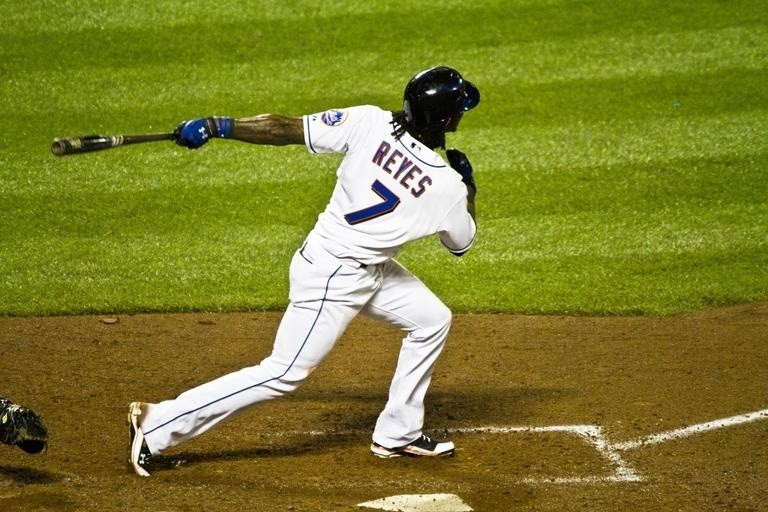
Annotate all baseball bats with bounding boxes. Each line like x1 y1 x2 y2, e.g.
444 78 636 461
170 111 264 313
51 131 178 156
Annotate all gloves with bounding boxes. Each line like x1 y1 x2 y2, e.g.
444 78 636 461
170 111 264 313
174 116 232 149
446 150 476 193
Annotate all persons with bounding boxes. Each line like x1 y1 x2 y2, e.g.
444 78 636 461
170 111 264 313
125 64 481 476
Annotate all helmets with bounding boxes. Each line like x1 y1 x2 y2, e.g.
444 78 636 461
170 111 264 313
404 66 480 127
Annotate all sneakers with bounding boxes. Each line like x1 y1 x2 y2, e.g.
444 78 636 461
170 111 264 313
370 435 455 459
127 402 154 477
